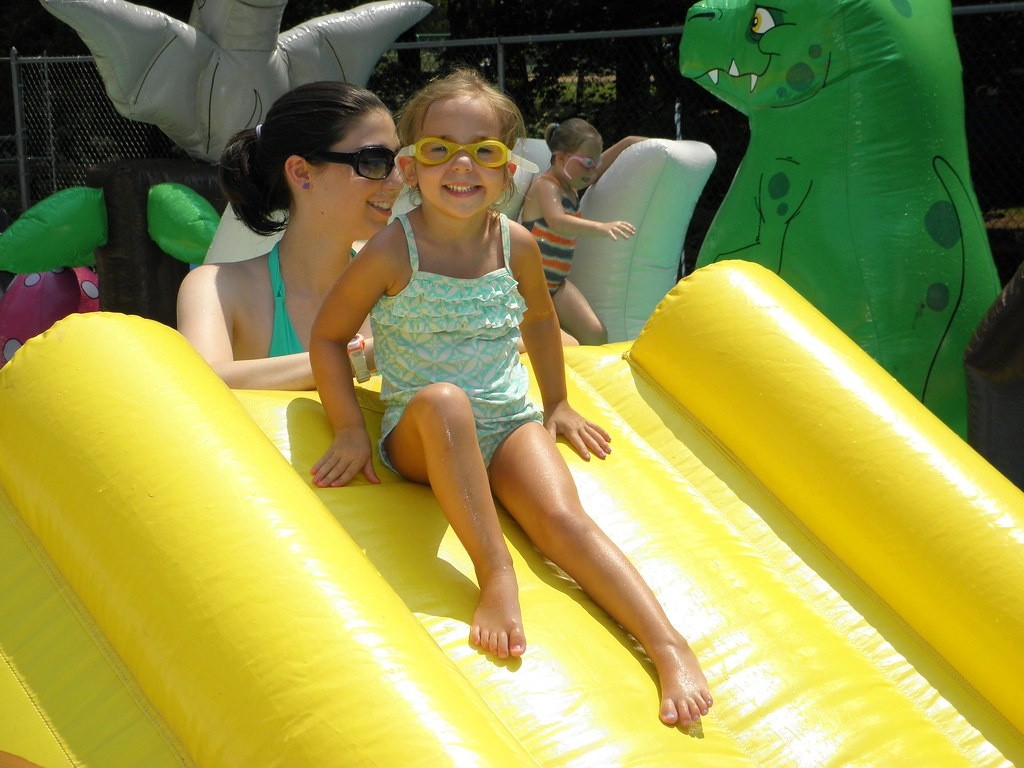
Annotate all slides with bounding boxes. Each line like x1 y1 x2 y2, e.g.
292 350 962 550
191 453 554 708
0 258 1024 768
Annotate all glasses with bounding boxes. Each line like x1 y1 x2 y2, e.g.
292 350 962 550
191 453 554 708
563 150 602 180
394 138 540 181
306 147 397 180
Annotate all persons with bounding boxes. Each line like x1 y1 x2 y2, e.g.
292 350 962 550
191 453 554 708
176 80 401 392
516 118 649 353
310 70 713 728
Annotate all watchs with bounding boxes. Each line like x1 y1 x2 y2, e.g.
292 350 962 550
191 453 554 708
346 333 372 384
